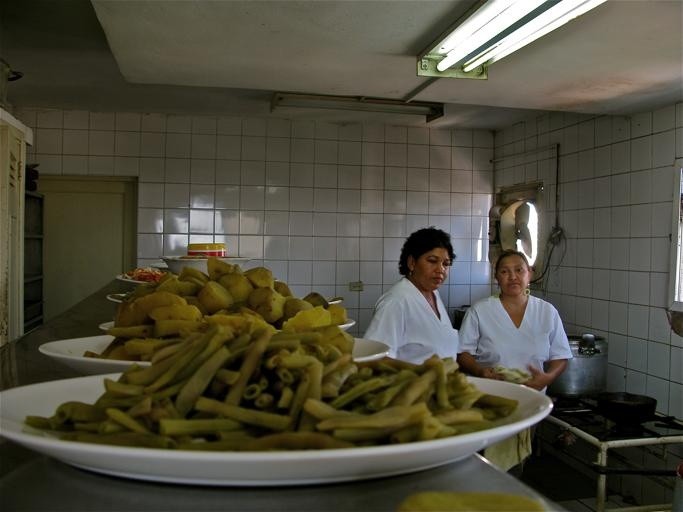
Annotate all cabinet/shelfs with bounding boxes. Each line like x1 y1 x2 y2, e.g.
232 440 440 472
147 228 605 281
21 190 45 333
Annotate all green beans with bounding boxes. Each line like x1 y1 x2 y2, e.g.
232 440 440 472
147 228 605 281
22 316 520 448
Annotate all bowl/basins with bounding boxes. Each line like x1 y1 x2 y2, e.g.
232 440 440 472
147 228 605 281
597 389 660 423
158 254 252 274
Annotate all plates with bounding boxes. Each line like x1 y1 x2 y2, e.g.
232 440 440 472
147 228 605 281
98 316 356 334
0 372 552 483
151 262 168 269
35 333 387 371
113 269 159 284
104 291 343 307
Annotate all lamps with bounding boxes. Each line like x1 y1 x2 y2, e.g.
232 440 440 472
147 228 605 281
416 1 609 84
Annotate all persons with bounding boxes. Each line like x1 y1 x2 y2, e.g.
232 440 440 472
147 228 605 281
458 250 572 478
362 226 458 360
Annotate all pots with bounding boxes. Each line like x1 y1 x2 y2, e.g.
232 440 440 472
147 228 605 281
544 332 608 398
